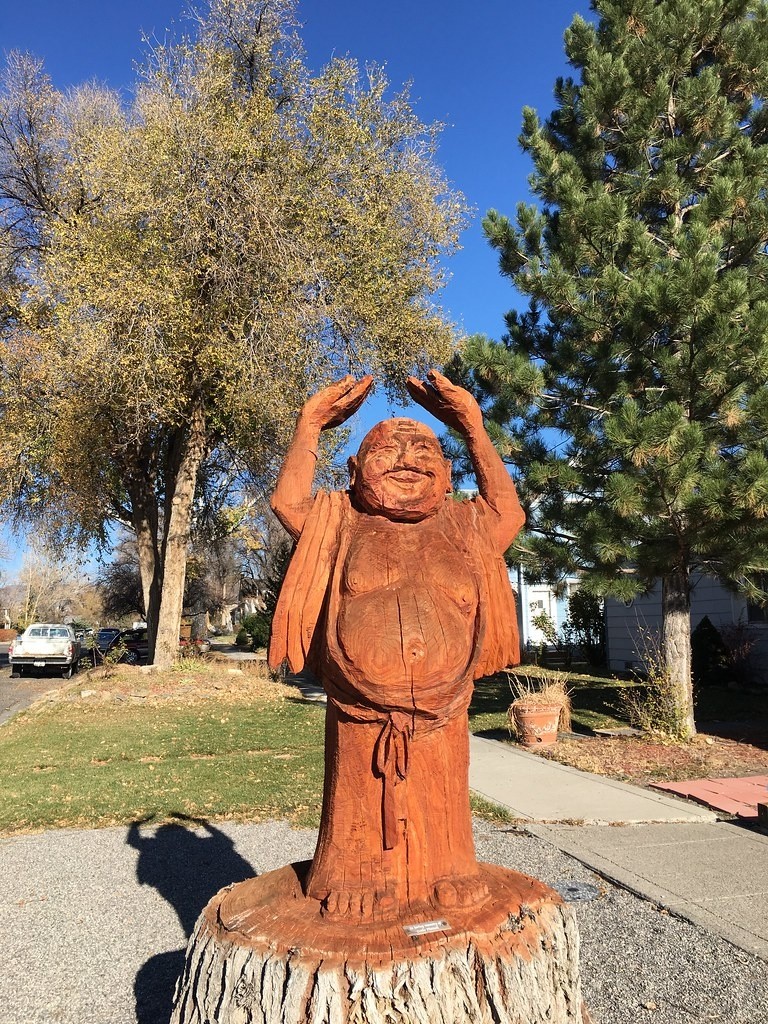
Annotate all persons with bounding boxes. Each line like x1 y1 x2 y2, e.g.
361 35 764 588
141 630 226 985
267 372 527 922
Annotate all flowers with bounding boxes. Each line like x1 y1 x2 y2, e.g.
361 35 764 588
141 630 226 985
195 640 204 646
179 640 189 647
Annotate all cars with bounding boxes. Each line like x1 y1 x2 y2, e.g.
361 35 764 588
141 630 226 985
104 628 211 665
84 628 94 635
75 629 86 638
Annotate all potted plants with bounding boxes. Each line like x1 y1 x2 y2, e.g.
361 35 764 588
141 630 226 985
505 670 576 747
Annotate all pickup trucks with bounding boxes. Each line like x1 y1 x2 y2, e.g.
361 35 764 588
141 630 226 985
8 622 82 680
95 628 120 643
